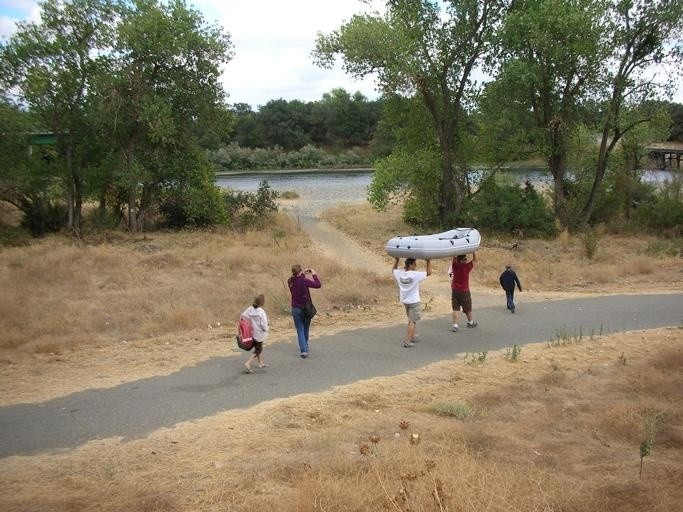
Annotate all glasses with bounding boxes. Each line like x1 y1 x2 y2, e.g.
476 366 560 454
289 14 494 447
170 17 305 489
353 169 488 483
461 258 467 261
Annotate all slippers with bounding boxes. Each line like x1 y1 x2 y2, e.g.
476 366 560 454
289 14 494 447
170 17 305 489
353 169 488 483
241 364 269 373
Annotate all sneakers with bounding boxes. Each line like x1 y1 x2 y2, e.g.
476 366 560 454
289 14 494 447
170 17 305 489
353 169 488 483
467 321 478 328
511 305 515 313
452 326 458 332
402 334 418 347
301 342 310 358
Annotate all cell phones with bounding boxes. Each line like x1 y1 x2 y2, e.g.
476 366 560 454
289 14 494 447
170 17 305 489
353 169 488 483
305 270 311 274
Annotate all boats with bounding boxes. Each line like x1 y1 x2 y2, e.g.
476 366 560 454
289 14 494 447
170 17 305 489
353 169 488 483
384 226 483 260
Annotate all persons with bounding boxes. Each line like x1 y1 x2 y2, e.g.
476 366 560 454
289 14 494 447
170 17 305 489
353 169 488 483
499 264 522 313
242 293 269 373
391 256 432 347
287 264 321 359
451 252 478 333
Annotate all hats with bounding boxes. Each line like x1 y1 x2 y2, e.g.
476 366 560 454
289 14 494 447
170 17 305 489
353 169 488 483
405 258 415 265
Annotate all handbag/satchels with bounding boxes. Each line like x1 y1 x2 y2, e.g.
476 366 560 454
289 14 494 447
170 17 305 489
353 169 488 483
237 318 255 351
302 302 316 322
